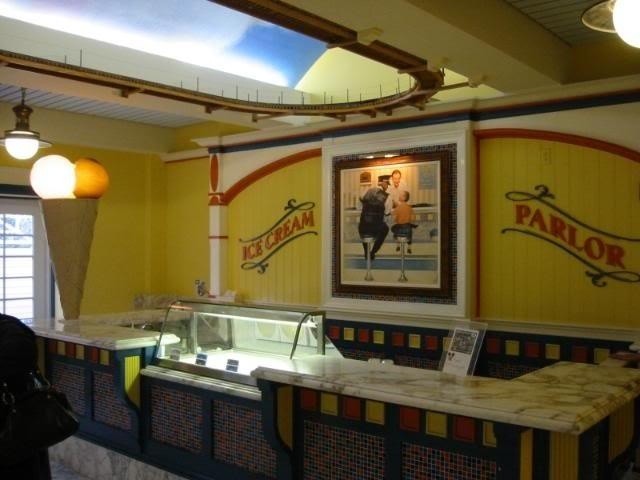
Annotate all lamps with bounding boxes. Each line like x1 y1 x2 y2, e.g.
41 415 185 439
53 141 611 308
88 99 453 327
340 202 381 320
581 1 640 51
29 154 110 319
0 87 53 161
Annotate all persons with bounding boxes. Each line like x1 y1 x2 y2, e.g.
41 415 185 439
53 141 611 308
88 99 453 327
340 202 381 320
356 174 394 263
390 189 417 256
385 168 404 209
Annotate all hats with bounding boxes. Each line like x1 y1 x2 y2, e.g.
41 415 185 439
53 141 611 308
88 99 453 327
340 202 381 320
378 175 392 185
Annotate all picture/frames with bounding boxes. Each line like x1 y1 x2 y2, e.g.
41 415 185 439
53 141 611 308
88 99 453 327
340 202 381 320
319 120 474 319
436 319 488 375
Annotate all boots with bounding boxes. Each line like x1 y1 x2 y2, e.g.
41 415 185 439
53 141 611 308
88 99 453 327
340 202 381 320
362 240 383 260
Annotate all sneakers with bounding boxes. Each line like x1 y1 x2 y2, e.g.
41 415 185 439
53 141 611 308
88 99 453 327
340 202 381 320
396 247 400 251
407 249 411 253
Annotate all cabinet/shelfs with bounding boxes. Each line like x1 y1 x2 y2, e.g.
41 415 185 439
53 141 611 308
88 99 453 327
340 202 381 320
139 298 325 402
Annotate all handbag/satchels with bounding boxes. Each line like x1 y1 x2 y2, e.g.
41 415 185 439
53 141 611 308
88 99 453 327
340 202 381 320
2 362 80 448
